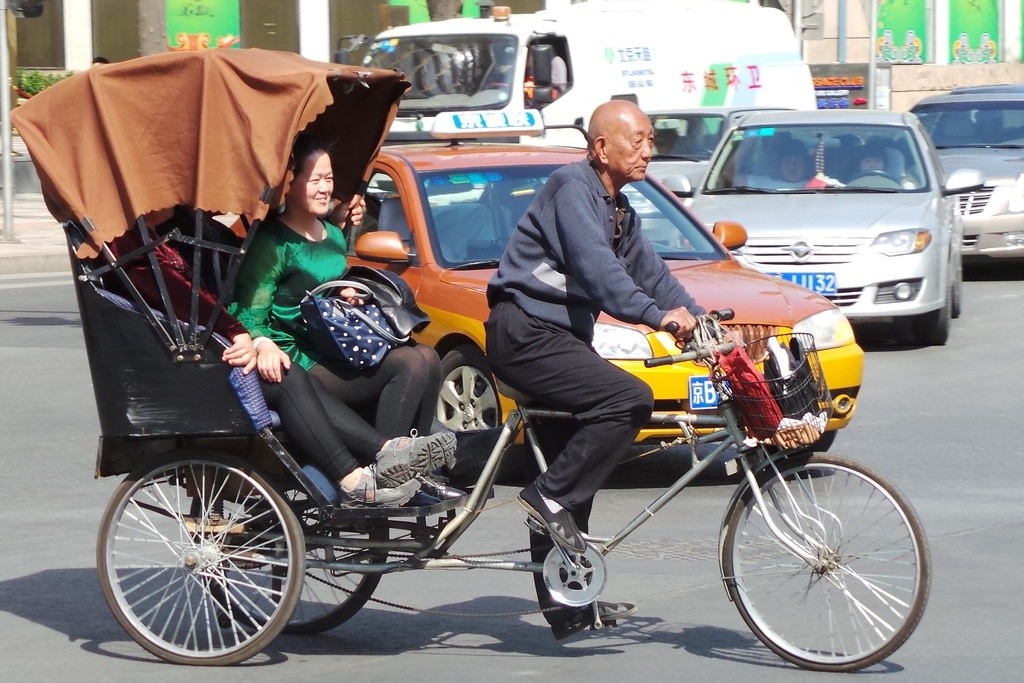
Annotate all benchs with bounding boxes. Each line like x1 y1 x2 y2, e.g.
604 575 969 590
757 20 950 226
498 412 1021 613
377 197 413 247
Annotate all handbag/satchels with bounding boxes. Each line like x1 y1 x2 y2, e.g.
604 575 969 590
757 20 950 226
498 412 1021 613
696 314 783 441
299 280 410 373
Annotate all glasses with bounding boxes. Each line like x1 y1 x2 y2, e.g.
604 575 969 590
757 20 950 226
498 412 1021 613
287 152 296 170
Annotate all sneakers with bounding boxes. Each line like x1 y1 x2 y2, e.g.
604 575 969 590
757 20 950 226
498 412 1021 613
376 428 458 488
339 463 422 509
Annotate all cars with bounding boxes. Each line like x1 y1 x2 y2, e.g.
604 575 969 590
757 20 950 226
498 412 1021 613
619 112 732 250
645 111 984 348
904 83 1024 267
360 110 866 484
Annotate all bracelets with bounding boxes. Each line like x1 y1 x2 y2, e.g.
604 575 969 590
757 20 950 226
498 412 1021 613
254 337 272 348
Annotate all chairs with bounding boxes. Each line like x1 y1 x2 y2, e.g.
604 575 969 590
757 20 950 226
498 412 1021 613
842 143 906 182
409 200 492 264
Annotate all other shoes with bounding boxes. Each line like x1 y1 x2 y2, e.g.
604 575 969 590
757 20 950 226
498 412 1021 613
516 482 587 553
553 601 637 640
408 471 468 506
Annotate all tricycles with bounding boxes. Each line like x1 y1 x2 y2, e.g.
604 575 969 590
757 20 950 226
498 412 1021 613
7 45 934 675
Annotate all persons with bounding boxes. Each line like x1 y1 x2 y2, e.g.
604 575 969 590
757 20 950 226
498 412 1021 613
722 128 812 188
484 99 751 640
856 145 920 190
227 142 467 507
154 193 519 488
526 51 568 96
97 208 457 509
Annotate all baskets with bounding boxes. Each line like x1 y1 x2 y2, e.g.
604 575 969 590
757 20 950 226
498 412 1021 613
710 332 834 449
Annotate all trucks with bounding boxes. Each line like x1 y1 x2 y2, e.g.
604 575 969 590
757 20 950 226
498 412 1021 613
328 0 821 210
